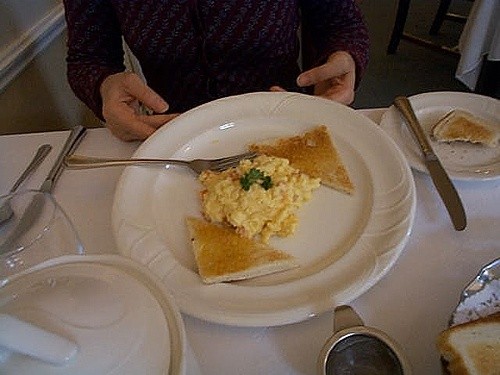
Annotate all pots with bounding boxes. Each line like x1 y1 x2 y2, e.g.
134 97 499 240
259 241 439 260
0 252 201 375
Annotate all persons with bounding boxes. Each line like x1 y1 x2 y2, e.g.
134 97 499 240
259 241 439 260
62 0 371 143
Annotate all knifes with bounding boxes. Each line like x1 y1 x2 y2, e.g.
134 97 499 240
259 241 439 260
392 97 467 232
0 125 87 254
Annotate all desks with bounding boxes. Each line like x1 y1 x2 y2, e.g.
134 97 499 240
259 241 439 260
0 108 500 375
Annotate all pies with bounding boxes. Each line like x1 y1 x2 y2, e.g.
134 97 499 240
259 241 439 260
250 125 355 194
187 217 300 284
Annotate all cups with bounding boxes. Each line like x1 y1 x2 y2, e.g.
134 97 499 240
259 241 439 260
0 190 85 282
317 307 411 375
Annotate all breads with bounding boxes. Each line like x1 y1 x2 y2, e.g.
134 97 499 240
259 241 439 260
431 109 500 148
436 311 500 375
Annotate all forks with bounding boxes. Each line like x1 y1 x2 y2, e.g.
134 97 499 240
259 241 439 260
63 153 256 175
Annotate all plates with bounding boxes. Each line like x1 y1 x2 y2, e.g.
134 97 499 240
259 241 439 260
381 91 500 183
110 91 416 326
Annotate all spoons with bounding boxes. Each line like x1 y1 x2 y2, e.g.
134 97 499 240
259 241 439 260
0 151 53 223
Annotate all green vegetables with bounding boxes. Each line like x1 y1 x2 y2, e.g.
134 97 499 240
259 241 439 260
241 167 274 192
